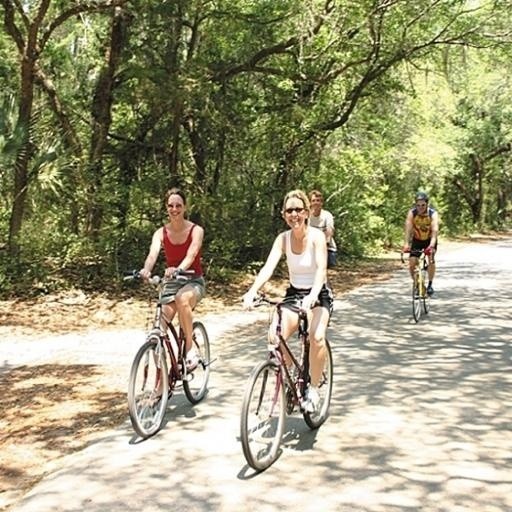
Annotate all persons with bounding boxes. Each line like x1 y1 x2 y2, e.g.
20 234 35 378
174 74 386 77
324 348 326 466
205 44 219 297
401 192 439 295
240 188 333 414
305 190 336 299
140 188 208 401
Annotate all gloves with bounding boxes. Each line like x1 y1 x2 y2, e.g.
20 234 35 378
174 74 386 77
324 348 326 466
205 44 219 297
425 247 431 255
403 246 410 252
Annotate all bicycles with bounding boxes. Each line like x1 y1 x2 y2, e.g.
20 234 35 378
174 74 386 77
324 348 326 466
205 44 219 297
295 283 334 337
118 264 217 441
399 246 436 323
234 291 335 472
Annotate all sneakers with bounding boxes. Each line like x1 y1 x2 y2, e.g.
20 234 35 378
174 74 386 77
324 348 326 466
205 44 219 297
427 287 433 294
185 342 199 370
301 387 319 413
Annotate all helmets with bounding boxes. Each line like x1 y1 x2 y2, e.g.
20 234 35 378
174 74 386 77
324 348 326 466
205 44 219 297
415 193 428 202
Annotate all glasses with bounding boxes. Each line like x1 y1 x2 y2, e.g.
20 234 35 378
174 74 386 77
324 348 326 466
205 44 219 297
284 208 304 213
